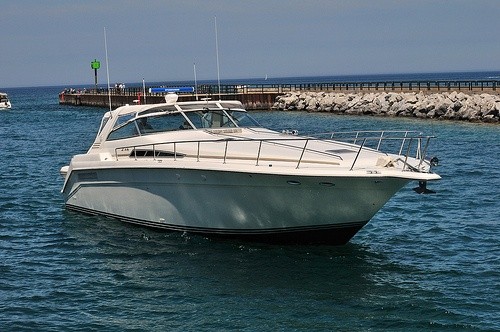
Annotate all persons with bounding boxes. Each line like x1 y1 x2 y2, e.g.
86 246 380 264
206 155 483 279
115 82 126 95
61 87 87 95
134 117 152 132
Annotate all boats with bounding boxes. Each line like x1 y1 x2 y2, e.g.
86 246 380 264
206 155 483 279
59 15 442 247
0 92 12 109
